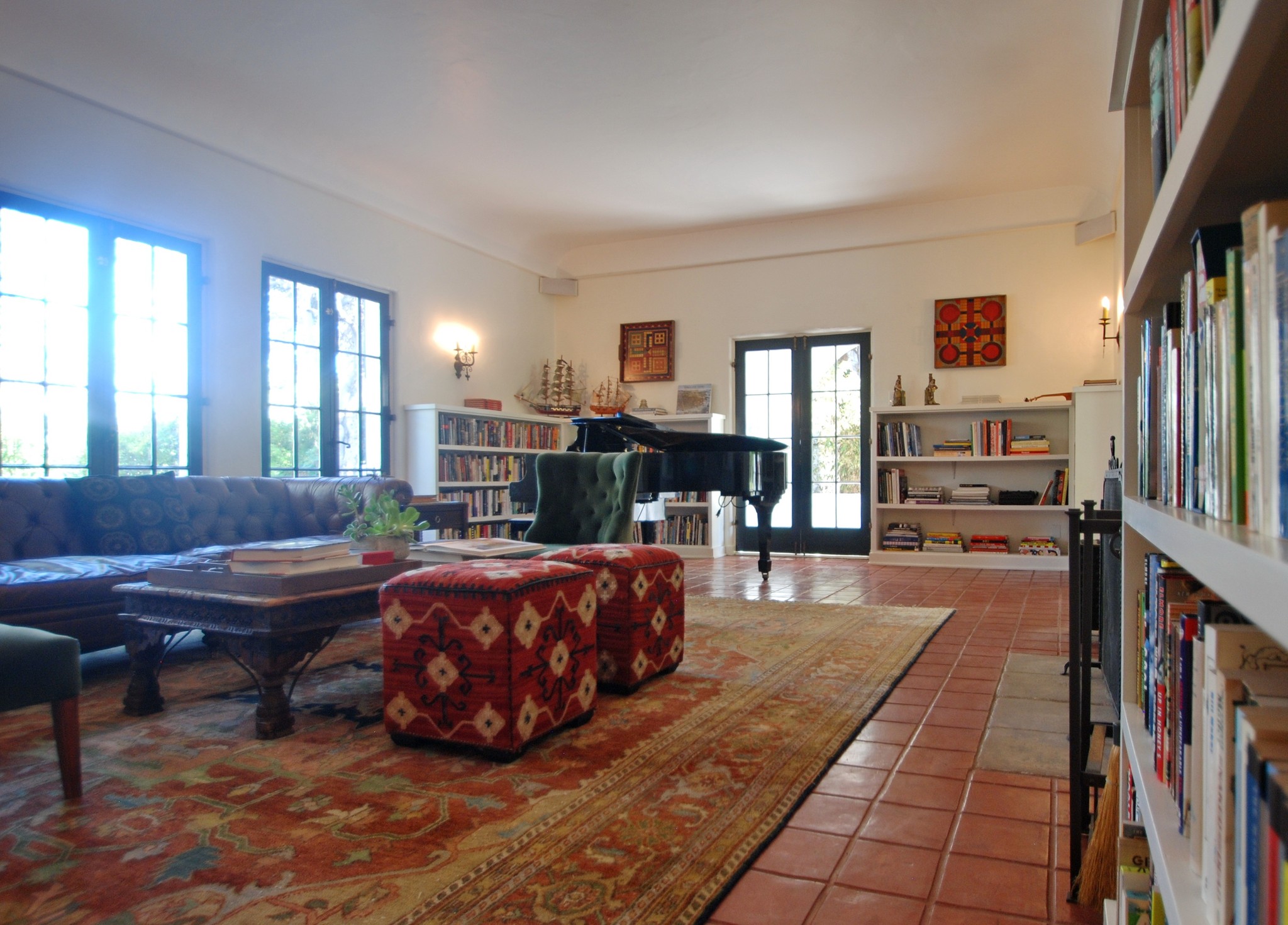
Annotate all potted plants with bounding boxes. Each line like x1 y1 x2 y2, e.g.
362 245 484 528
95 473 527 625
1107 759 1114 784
338 483 431 560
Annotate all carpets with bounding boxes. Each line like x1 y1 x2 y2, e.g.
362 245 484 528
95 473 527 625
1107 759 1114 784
0 592 957 925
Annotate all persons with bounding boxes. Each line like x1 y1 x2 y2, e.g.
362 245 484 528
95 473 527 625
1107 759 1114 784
924 379 940 405
893 380 902 406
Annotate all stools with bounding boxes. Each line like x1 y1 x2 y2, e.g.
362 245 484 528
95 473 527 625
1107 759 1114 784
527 542 686 699
377 558 599 766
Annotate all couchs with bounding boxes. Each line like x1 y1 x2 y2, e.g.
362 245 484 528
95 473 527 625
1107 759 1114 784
0 471 414 713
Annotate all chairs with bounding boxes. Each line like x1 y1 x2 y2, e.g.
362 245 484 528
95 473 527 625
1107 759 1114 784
0 623 83 799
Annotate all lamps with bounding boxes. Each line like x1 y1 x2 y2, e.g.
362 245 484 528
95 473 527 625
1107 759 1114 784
439 322 480 381
1097 296 1120 358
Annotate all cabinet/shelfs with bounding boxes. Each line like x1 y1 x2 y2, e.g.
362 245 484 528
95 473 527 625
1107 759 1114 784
561 412 727 560
868 0 1288 925
403 403 562 543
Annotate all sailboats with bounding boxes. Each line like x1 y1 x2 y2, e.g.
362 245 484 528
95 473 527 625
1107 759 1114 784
589 376 632 414
514 355 586 416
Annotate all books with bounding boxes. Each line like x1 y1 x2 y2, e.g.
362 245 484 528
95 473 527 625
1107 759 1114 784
224 538 362 578
968 534 1009 555
1017 536 1061 556
625 440 709 545
932 418 1050 456
405 413 561 562
961 395 1002 404
875 418 924 458
1082 379 1118 386
877 468 1069 506
1113 0 1288 925
881 522 967 557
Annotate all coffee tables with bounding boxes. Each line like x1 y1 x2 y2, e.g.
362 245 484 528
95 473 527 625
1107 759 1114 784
106 535 548 741
